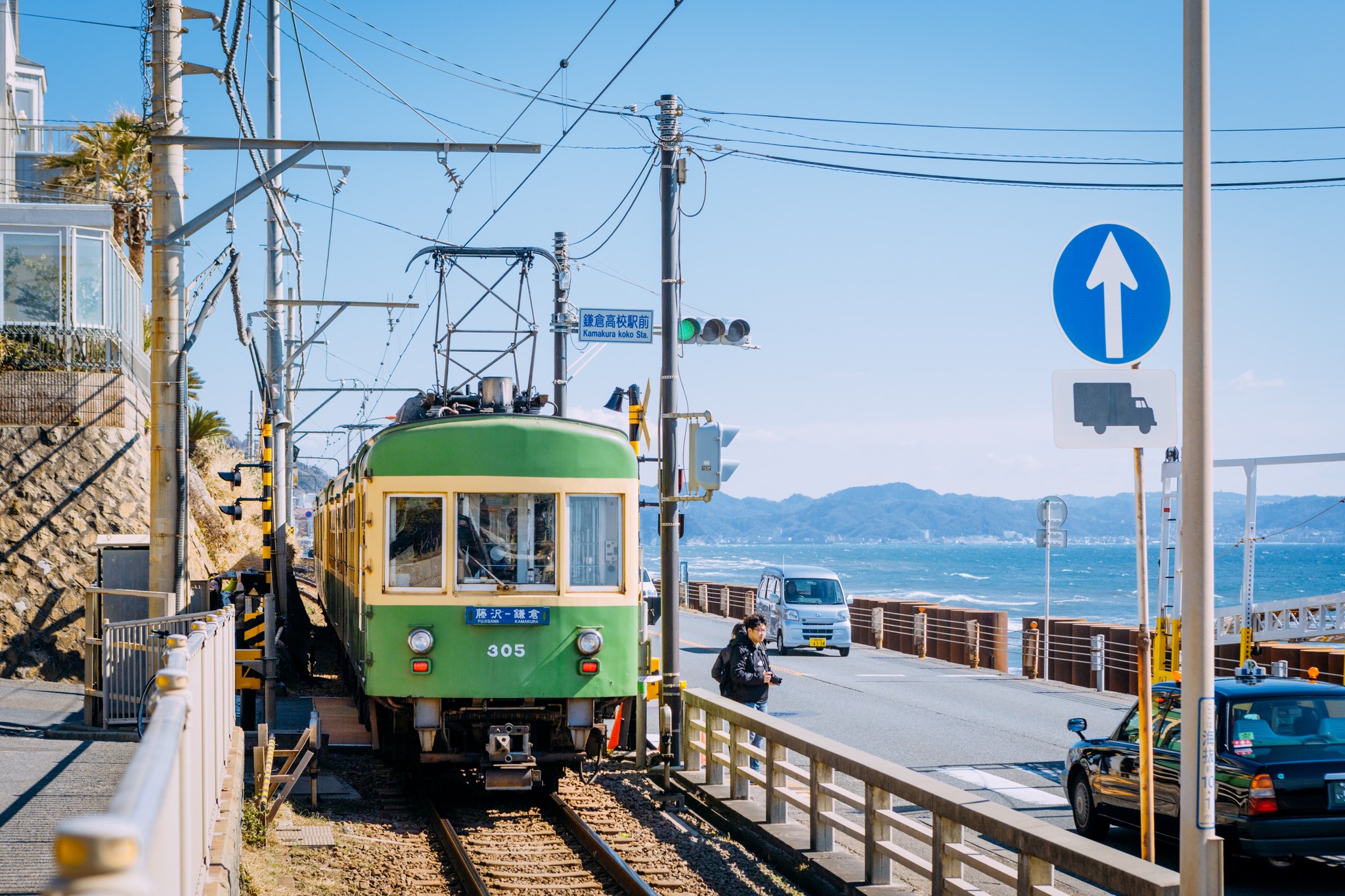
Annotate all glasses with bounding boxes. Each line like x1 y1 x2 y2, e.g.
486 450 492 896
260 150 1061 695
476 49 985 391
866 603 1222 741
750 627 768 633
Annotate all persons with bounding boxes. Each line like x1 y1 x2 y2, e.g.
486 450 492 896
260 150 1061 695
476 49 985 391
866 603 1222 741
506 511 555 576
711 622 748 753
730 612 777 785
208 570 239 593
785 581 804 601
210 585 220 611
391 497 481 577
210 579 231 608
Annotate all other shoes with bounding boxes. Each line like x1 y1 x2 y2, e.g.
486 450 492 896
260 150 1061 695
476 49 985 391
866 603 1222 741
750 780 757 785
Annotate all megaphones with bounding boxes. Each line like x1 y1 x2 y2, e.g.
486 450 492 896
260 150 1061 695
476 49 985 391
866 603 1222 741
603 386 624 413
273 409 291 430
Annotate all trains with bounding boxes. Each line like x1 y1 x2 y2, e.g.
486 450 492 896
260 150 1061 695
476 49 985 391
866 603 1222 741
310 248 652 798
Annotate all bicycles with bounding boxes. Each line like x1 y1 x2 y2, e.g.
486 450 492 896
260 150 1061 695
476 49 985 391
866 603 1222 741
137 630 175 738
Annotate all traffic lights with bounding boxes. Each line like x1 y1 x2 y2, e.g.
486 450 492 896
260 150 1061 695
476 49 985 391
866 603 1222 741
216 467 242 491
218 500 243 524
698 421 740 494
677 317 752 348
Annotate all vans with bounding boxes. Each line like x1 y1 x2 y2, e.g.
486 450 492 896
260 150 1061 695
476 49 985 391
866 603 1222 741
755 565 854 656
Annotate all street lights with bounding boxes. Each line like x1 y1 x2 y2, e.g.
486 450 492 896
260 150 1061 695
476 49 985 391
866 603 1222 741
602 385 645 477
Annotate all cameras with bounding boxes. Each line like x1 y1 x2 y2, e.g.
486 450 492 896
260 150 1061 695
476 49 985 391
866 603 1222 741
768 673 783 686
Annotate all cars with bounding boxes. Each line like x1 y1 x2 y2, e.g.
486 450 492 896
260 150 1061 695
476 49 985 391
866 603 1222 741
1063 662 1345 879
638 567 659 598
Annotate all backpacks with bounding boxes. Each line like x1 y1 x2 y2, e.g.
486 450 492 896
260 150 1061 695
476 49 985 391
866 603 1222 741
719 644 745 697
